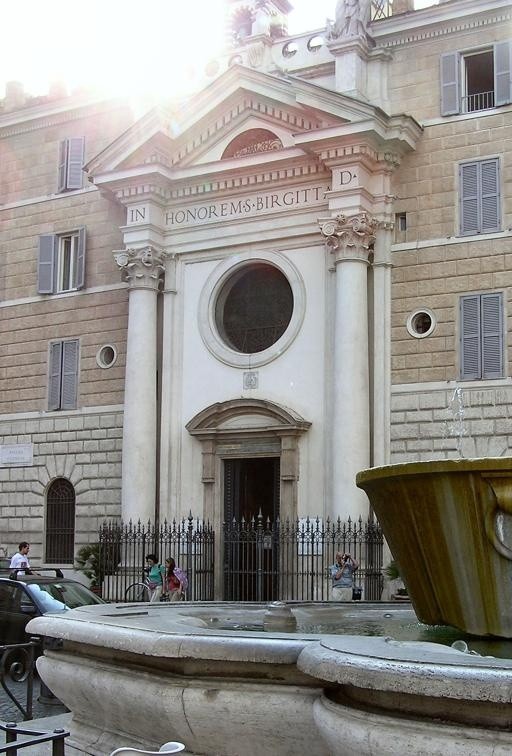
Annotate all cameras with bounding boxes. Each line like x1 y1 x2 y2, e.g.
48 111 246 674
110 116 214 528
343 553 348 562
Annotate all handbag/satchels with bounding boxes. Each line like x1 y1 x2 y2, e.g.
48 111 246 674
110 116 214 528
174 576 180 585
148 582 157 589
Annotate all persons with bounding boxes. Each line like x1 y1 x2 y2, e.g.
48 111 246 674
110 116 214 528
9 541 38 576
165 557 183 602
145 554 166 602
330 551 358 601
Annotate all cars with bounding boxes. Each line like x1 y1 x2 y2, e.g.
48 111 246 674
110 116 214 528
1 566 107 667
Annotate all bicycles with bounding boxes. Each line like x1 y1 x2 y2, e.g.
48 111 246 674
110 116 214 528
125 567 189 603
